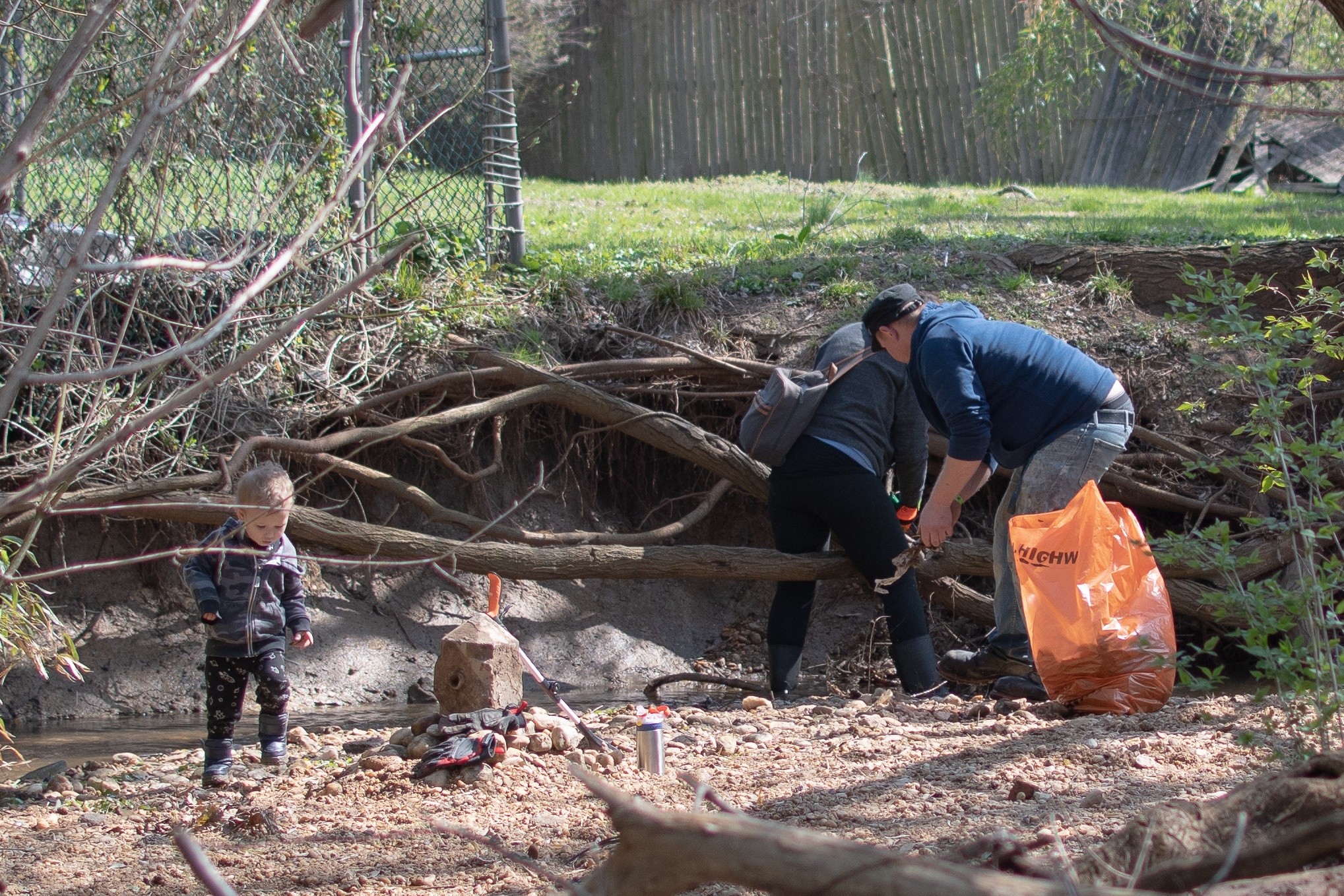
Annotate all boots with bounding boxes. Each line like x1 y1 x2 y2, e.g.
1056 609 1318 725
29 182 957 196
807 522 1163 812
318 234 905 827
888 636 976 700
259 709 289 762
203 737 233 785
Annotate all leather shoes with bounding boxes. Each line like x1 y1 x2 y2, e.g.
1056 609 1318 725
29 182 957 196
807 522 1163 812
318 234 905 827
936 645 1042 685
992 677 1047 704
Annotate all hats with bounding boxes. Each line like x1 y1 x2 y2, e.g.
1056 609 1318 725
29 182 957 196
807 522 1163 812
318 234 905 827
862 282 921 351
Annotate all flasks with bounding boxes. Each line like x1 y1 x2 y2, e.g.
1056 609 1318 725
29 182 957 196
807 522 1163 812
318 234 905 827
635 705 665 775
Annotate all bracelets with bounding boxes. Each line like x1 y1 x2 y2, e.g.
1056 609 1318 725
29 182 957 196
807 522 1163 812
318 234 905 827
954 495 964 504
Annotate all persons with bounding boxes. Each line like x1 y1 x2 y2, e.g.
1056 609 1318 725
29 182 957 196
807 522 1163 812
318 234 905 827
183 460 314 784
862 283 1135 700
766 321 975 701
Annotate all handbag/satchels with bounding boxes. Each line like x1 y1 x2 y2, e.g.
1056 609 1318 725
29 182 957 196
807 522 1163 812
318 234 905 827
740 361 839 466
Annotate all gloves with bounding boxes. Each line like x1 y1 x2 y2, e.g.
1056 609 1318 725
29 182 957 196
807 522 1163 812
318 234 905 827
439 700 527 734
411 734 497 777
894 501 917 531
